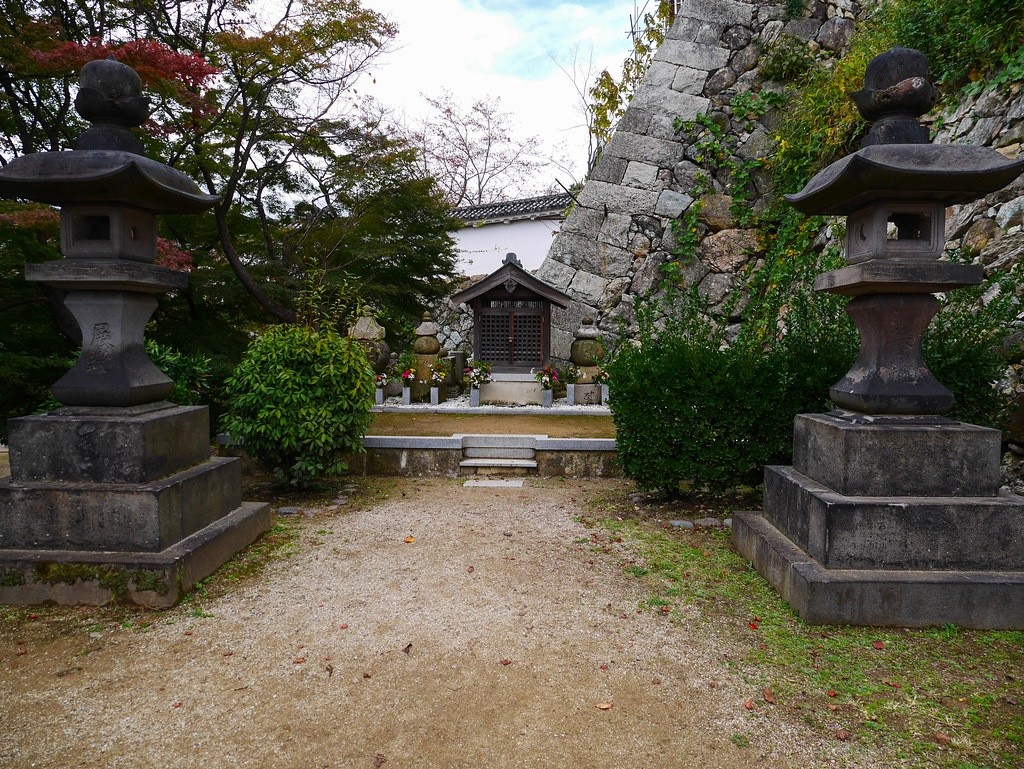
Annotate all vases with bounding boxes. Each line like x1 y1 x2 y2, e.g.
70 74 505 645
376 388 384 403
470 389 480 406
430 387 439 405
403 387 410 405
567 383 575 405
543 390 553 408
601 384 609 406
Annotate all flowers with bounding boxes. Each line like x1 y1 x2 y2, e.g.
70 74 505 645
463 360 497 390
376 373 387 388
401 368 416 386
592 371 609 385
419 358 447 386
559 367 586 384
534 367 558 391
